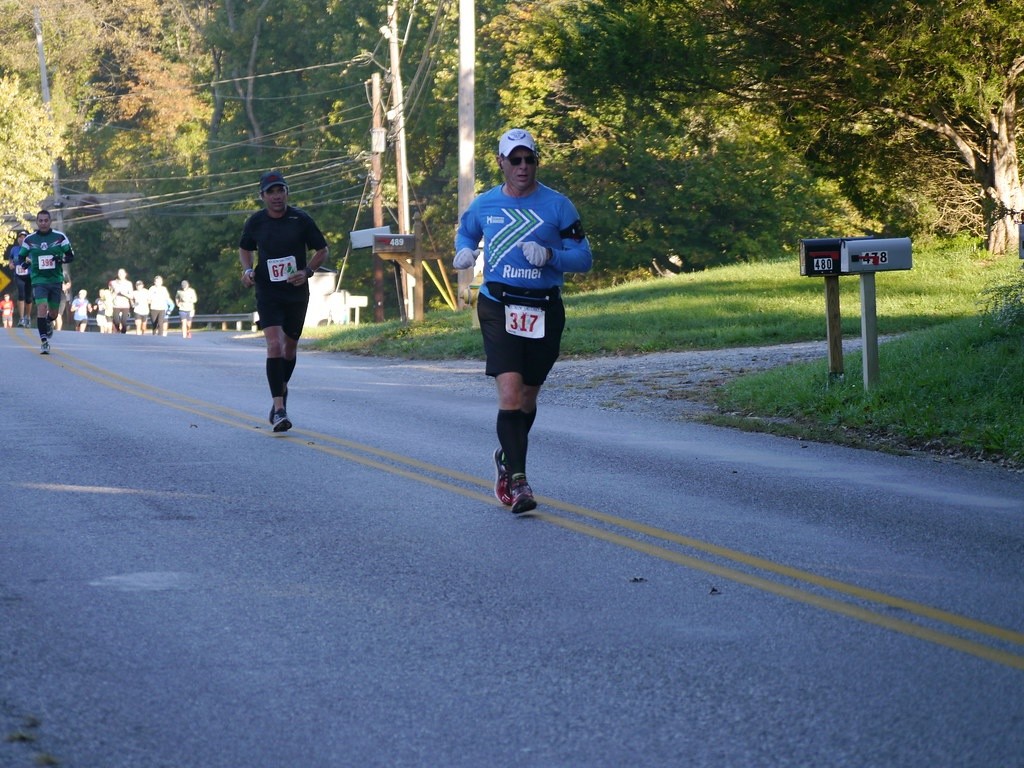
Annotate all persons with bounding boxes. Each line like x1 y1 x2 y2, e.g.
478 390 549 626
0 293 15 327
50 267 72 332
20 210 74 354
70 289 92 332
453 127 592 512
10 230 34 329
239 172 329 431
176 280 197 339
93 268 174 337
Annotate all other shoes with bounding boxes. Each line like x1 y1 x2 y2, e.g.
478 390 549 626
24 315 31 328
18 319 25 327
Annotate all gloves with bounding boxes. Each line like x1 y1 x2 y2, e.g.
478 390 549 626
517 241 547 267
453 248 480 269
51 255 63 264
21 261 30 269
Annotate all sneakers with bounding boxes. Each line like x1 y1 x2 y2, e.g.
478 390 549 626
493 446 512 506
40 340 50 354
509 474 537 514
273 409 292 432
269 404 275 424
46 317 53 339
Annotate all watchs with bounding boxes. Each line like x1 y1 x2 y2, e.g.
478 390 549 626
304 266 314 277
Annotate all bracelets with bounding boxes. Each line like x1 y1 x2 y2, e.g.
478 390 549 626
244 268 253 274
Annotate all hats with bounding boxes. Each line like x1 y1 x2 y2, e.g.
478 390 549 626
260 171 289 191
498 129 535 158
18 231 27 237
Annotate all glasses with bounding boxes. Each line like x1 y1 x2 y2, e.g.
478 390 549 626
503 155 536 165
